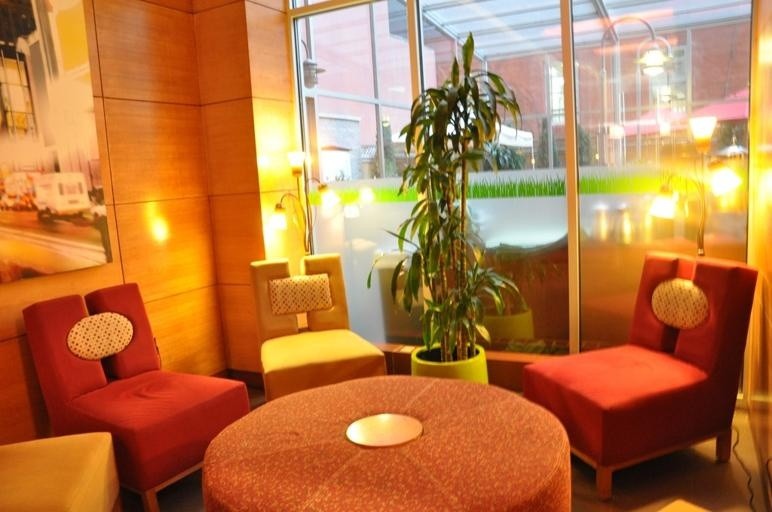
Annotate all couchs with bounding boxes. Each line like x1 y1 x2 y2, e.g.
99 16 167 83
20 283 250 512
0 432 122 511
248 255 386 402
522 251 759 501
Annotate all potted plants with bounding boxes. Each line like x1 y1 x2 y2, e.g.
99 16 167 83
384 31 523 384
472 239 565 342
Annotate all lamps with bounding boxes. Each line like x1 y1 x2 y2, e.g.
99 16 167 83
647 118 743 255
273 152 341 258
301 39 318 89
